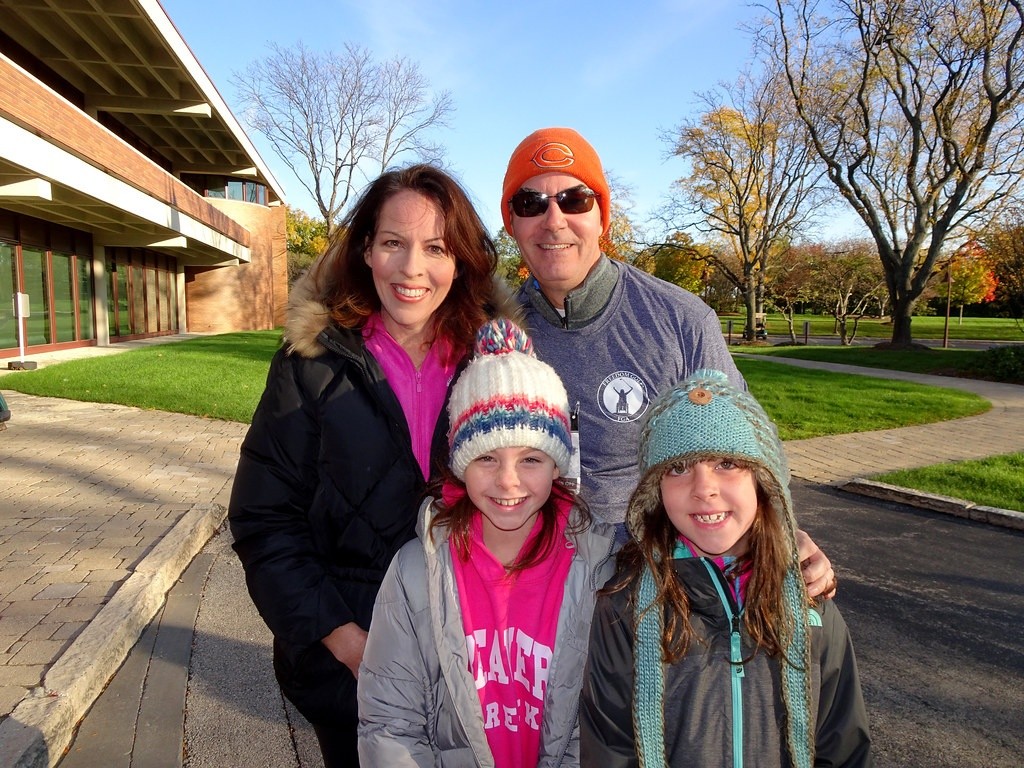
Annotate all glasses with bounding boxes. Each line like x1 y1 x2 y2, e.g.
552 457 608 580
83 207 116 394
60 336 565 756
508 183 600 217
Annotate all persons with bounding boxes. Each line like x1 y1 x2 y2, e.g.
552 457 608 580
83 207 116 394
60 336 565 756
230 164 527 768
577 369 872 768
355 320 623 768
502 126 837 599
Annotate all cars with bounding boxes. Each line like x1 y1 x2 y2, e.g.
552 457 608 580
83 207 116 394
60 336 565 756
743 324 767 339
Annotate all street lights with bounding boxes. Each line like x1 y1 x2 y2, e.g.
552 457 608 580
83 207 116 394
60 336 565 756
941 258 955 348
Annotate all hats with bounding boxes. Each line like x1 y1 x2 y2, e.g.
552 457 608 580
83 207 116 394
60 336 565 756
501 127 610 238
633 366 812 768
446 315 574 485
